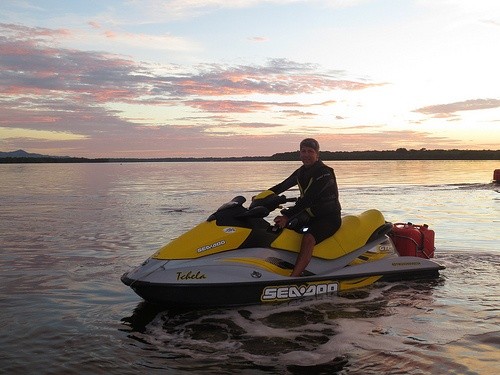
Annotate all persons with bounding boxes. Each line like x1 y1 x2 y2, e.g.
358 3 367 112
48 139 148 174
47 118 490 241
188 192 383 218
249 138 342 277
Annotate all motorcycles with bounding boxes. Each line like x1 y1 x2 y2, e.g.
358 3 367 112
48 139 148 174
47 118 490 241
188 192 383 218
121 190 446 307
